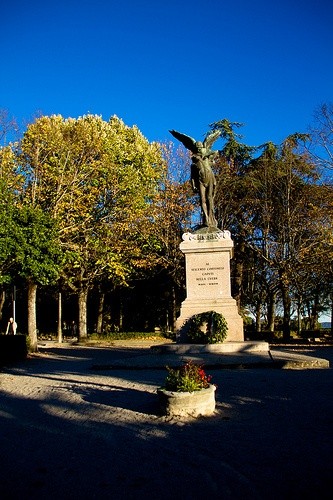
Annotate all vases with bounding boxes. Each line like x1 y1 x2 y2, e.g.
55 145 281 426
158 383 216 417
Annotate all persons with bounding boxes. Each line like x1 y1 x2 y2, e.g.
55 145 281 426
70 320 77 339
5 317 17 335
62 321 68 339
189 140 217 192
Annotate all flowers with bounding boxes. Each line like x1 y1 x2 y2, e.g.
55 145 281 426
156 358 212 395
